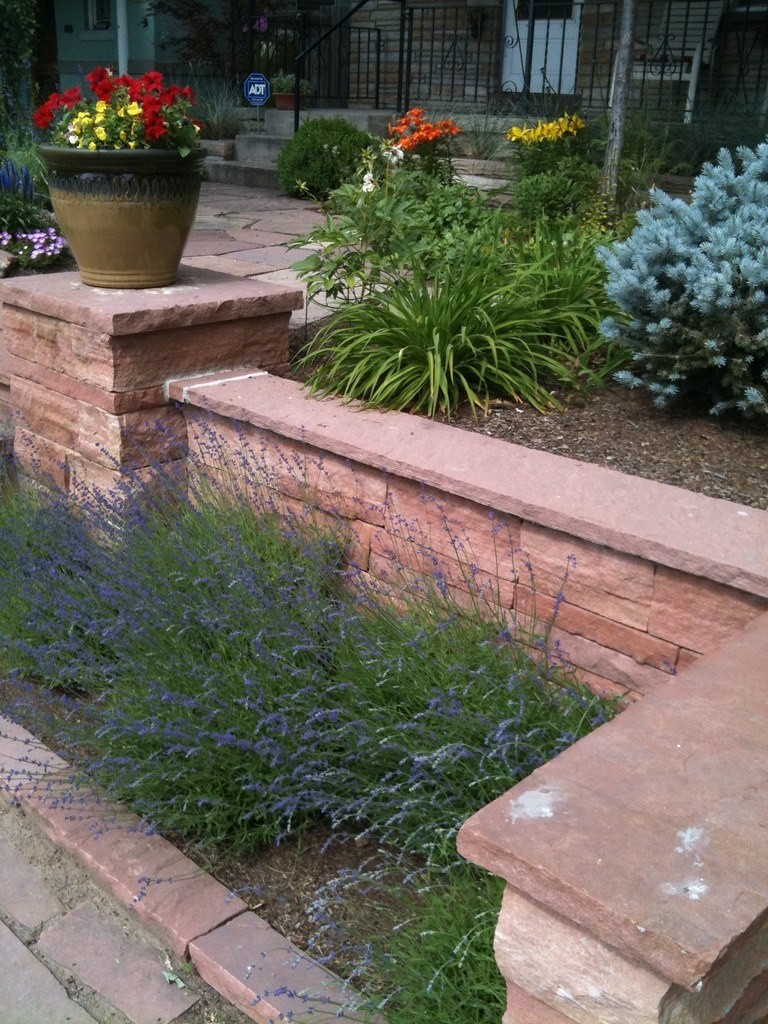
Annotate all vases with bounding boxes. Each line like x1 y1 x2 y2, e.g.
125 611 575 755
37 142 206 289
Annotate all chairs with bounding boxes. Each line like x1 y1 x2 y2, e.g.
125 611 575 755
609 0 727 125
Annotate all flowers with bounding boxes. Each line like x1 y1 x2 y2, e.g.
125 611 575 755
32 67 207 158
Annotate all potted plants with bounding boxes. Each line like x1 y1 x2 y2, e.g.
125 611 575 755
270 68 312 111
